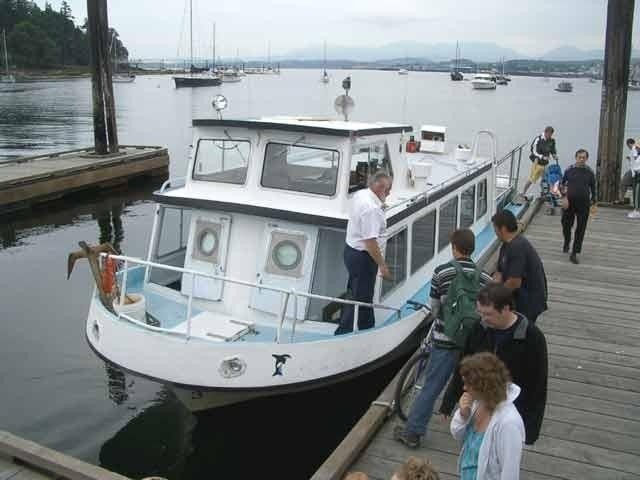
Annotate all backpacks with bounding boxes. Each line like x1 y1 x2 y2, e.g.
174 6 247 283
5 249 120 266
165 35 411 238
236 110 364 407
442 260 484 350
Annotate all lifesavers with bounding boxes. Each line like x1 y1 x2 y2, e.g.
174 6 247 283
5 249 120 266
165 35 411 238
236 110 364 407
102 256 113 292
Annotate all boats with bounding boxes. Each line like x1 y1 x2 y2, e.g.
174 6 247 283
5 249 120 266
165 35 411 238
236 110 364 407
628 80 640 90
79 94 532 412
173 61 282 89
318 72 330 84
110 74 136 84
555 83 573 93
450 69 512 91
398 69 409 75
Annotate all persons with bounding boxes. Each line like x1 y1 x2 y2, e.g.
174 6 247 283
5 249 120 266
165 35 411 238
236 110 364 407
558 149 596 264
439 281 548 445
334 170 392 335
613 137 640 219
491 209 547 324
518 126 559 198
390 456 444 480
450 351 526 480
393 228 484 448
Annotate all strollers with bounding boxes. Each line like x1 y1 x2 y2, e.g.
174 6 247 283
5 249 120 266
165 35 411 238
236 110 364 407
535 155 566 216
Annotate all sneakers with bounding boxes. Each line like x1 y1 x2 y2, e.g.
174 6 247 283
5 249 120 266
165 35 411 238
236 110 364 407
394 426 422 448
624 211 640 219
564 242 569 253
570 256 578 264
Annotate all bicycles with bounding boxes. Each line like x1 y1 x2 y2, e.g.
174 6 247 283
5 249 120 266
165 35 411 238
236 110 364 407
392 299 442 422
